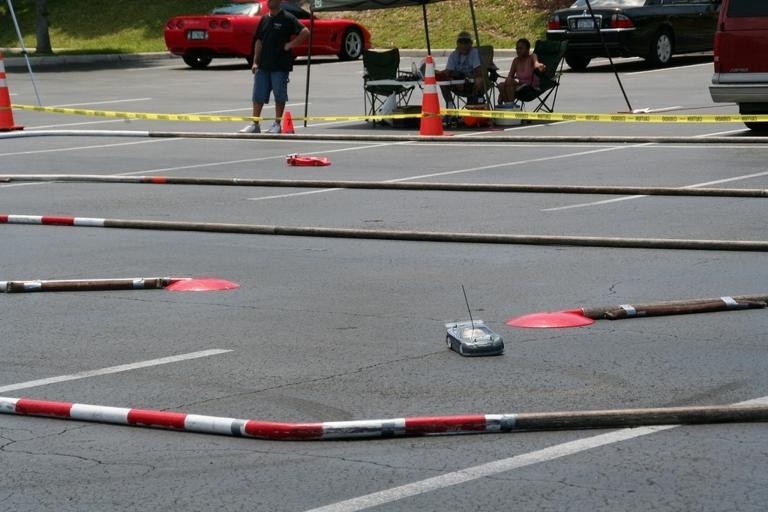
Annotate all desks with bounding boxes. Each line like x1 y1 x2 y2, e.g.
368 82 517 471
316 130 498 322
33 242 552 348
399 78 475 128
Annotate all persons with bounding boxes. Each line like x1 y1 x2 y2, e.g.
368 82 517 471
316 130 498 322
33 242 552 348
440 31 487 110
238 1 311 135
496 38 547 105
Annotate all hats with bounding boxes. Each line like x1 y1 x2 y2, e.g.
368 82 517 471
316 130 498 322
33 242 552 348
455 32 472 39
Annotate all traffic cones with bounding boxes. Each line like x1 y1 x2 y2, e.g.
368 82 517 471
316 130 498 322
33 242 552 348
281 111 295 134
418 54 447 136
0 49 25 133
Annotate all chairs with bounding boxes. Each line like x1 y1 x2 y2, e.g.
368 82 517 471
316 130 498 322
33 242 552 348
495 40 570 121
446 46 495 108
360 46 415 127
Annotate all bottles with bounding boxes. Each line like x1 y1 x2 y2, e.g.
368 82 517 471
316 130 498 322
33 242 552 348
412 61 417 77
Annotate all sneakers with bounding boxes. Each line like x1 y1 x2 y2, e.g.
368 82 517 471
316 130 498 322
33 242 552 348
240 122 261 132
265 121 281 133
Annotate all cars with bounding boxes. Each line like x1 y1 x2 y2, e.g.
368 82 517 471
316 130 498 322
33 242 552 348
708 1 768 133
547 0 721 72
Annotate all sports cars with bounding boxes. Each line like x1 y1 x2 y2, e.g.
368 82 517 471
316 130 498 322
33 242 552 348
164 1 372 70
